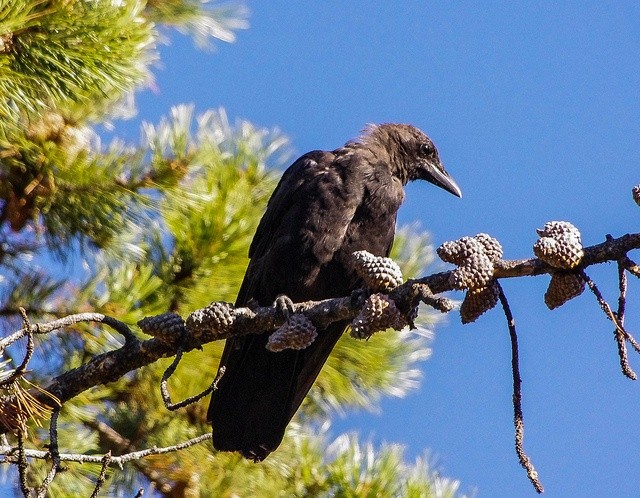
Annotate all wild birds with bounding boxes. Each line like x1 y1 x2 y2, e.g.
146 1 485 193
206 122 462 462
